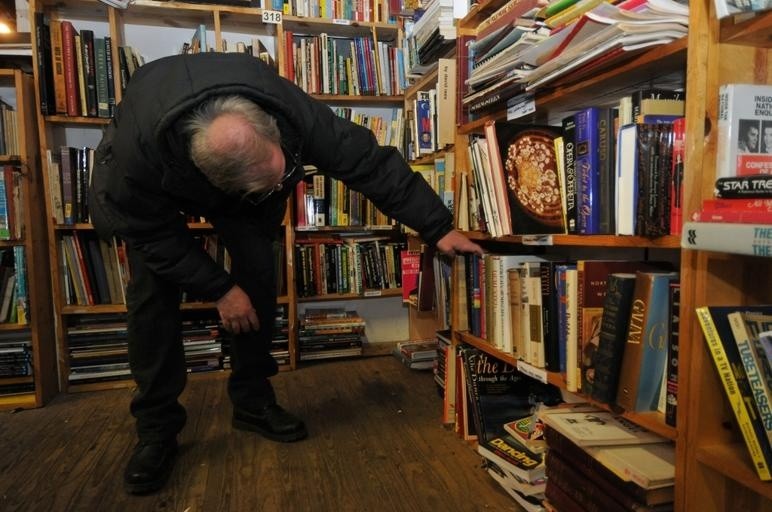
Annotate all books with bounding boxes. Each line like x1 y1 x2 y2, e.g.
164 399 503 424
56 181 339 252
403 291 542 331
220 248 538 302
296 308 366 361
452 0 691 128
190 227 233 275
0 245 30 326
294 231 410 299
453 254 680 427
221 38 276 67
334 107 406 152
455 344 676 512
283 30 405 97
0 330 34 396
407 150 456 223
271 235 287 296
0 160 25 242
270 0 401 26
294 174 420 236
396 0 457 90
56 229 131 306
63 305 290 386
0 99 20 156
694 306 772 482
0 0 32 50
392 338 438 370
116 46 147 96
33 12 117 120
46 145 96 225
680 84 772 259
400 242 453 328
401 58 458 164
182 24 216 55
453 68 686 238
433 330 452 400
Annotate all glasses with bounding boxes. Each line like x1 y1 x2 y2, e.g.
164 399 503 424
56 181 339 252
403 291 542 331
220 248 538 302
229 138 301 206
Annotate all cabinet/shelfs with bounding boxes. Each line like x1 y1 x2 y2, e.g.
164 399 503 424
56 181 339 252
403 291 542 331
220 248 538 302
258 16 471 363
0 67 57 413
695 0 772 512
444 0 696 512
399 46 459 348
27 0 296 394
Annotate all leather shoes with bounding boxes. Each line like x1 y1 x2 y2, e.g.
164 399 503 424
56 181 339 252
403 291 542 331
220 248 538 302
232 401 307 444
124 430 178 495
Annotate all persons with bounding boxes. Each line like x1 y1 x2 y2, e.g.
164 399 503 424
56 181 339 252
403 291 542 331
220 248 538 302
85 51 486 497
